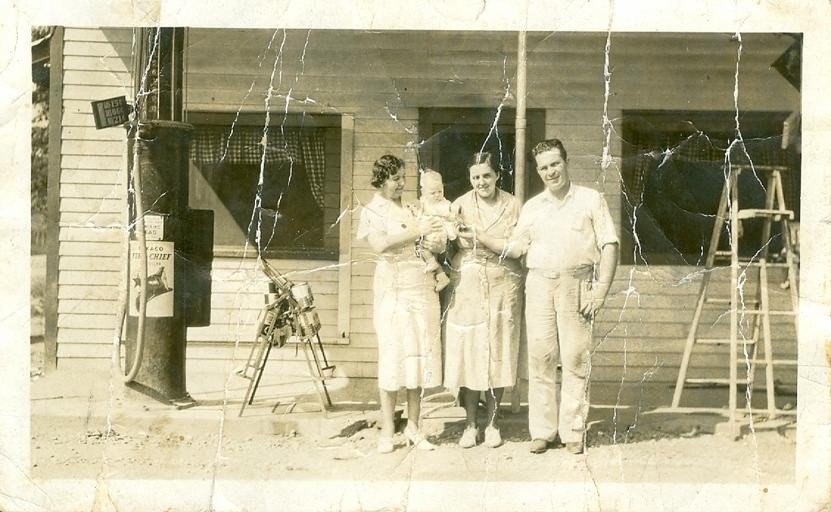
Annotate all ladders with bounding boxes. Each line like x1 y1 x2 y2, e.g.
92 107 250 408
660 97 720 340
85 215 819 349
672 164 799 427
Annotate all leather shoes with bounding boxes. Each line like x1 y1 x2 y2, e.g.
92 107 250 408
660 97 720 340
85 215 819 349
530 437 548 452
565 440 584 455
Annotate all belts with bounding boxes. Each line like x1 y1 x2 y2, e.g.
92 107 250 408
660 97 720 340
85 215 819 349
543 267 590 280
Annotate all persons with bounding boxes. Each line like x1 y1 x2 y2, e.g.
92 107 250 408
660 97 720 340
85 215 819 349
410 166 457 293
443 152 525 449
356 154 444 453
456 137 620 456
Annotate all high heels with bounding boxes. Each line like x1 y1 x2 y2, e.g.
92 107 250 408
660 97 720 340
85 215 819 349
378 435 394 452
403 420 437 450
485 424 502 446
461 425 481 448
